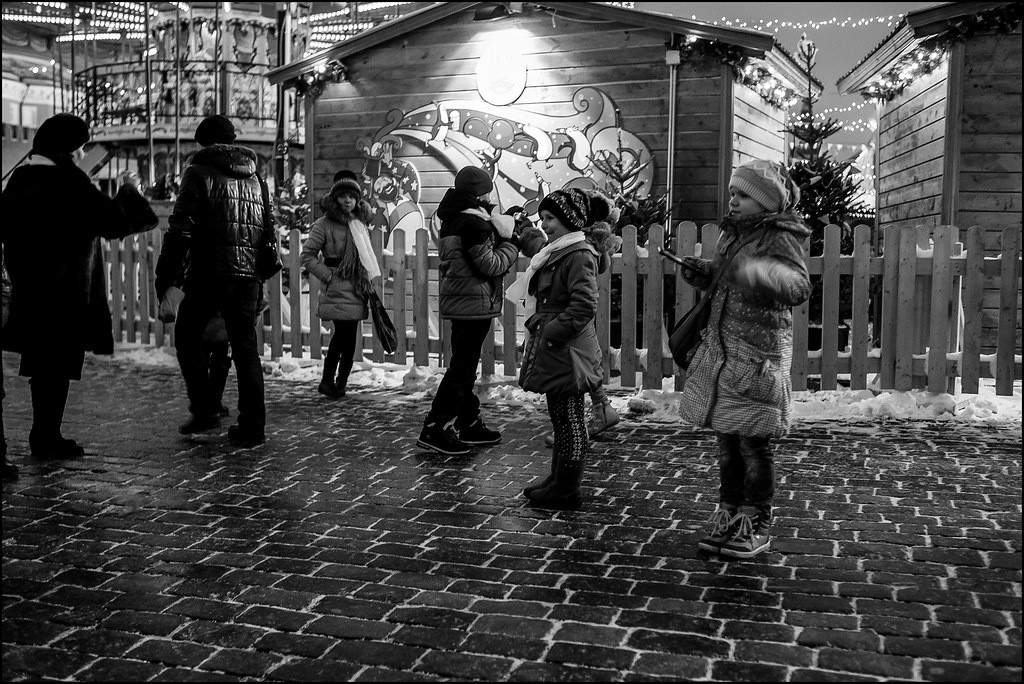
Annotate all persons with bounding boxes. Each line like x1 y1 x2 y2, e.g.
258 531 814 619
414 167 518 455
0 113 159 462
678 159 810 555
517 186 621 508
298 171 376 400
150 115 282 459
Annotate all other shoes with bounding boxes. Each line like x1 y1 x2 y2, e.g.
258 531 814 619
177 414 220 436
29 432 84 460
227 424 266 444
220 405 230 418
0 456 19 478
336 379 346 398
318 380 339 399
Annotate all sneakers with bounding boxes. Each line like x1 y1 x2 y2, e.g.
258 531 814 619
698 503 736 552
720 506 772 559
586 400 619 435
416 418 470 456
459 416 502 444
545 432 555 445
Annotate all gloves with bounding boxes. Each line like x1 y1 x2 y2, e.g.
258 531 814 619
154 275 180 302
503 205 524 217
506 234 527 252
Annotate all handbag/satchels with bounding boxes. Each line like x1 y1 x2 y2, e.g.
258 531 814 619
668 298 716 371
368 288 398 354
253 172 282 279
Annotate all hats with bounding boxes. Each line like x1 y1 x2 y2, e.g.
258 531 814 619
194 115 237 147
455 165 493 196
32 113 91 154
538 187 610 232
330 169 362 202
729 159 800 214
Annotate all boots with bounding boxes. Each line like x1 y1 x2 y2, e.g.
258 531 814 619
523 444 556 498
530 459 584 510
